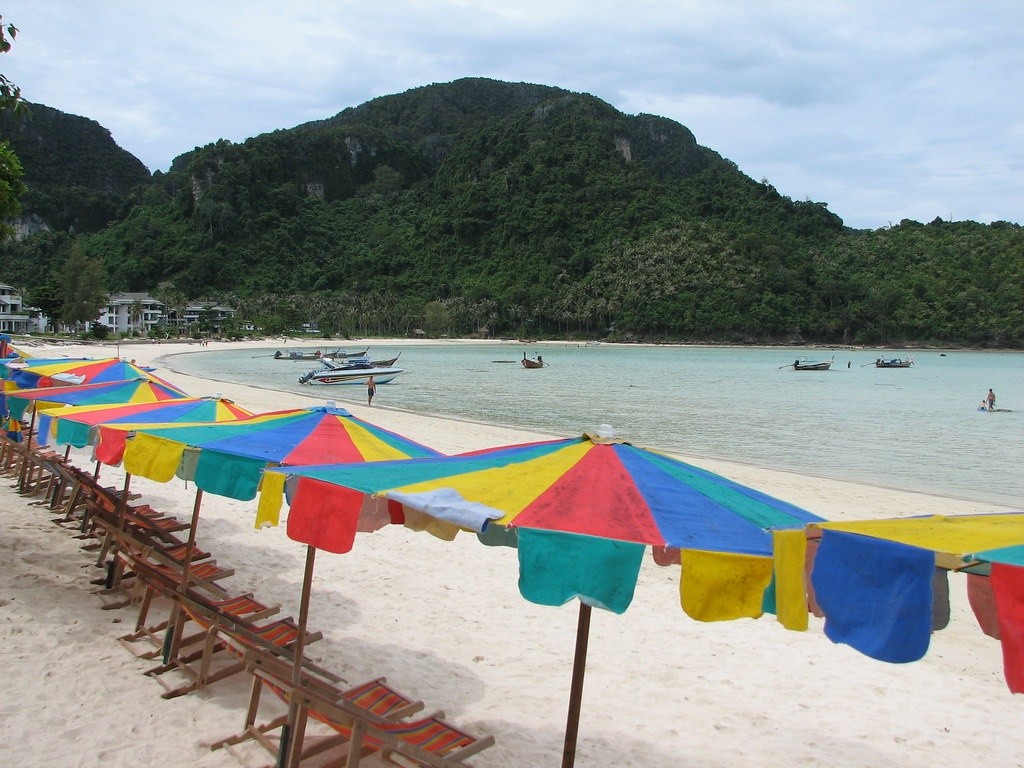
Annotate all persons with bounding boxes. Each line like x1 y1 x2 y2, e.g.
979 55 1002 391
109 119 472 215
152 337 287 344
364 376 376 405
795 354 996 411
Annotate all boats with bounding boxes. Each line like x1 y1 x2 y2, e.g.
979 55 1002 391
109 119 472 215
274 346 404 386
875 354 915 368
792 356 835 371
521 350 544 368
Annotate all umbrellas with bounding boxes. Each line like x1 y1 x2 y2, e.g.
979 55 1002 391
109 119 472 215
0 340 460 768
255 424 833 768
760 512 1024 696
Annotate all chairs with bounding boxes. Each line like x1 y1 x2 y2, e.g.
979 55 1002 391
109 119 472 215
0 416 495 768
